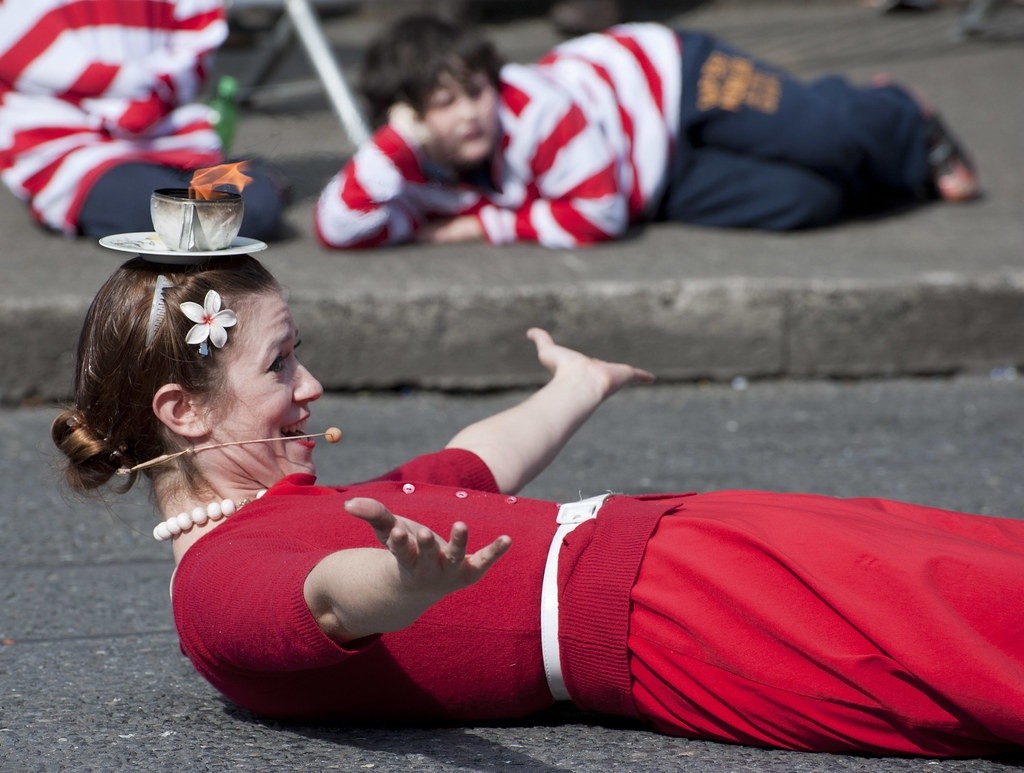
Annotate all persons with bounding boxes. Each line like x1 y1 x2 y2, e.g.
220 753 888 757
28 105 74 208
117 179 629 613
1 0 294 238
313 12 977 252
49 253 1024 764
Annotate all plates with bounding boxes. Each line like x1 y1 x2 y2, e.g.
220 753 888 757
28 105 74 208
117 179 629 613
99 232 268 264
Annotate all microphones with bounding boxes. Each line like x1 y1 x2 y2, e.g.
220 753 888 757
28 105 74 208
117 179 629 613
192 427 343 453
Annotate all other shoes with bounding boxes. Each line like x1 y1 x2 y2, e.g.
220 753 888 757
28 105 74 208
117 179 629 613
925 102 975 203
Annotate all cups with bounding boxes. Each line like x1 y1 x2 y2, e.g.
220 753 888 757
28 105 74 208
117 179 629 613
152 188 243 252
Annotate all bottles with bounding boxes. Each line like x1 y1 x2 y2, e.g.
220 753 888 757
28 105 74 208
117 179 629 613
205 74 238 159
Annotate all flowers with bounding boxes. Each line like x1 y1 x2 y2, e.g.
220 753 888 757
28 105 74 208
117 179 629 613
180 290 237 348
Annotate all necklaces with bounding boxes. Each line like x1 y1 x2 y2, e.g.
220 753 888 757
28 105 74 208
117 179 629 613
153 490 266 542
